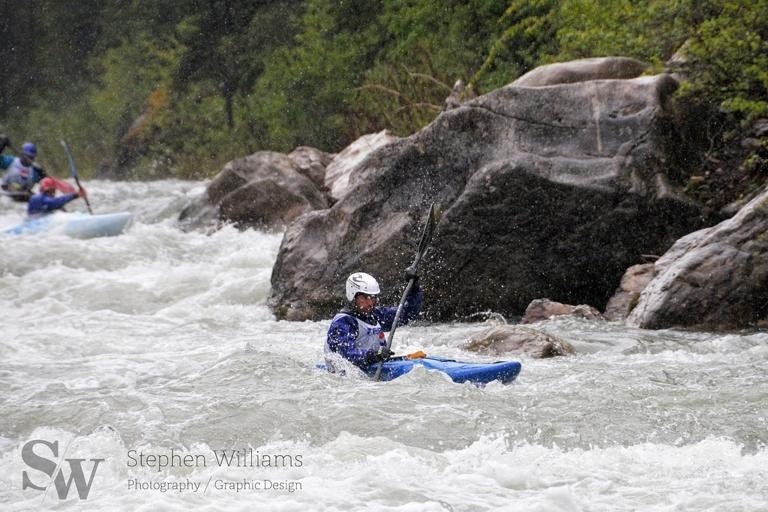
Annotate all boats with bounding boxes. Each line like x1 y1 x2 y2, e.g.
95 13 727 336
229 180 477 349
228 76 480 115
2 211 134 238
0 189 35 204
318 353 522 388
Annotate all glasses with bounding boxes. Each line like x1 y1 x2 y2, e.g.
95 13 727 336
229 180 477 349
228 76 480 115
356 292 377 301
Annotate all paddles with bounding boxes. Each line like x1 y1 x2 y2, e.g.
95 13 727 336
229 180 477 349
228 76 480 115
7 141 75 194
57 136 95 216
373 203 436 380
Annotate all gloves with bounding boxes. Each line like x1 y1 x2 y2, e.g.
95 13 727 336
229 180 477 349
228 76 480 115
366 348 395 363
404 266 419 292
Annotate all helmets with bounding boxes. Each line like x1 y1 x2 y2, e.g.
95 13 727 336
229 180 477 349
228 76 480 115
346 273 381 303
39 177 57 194
22 143 36 158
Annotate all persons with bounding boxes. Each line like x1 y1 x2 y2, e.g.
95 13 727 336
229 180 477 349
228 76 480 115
27 176 85 216
323 265 425 377
0 135 46 192
